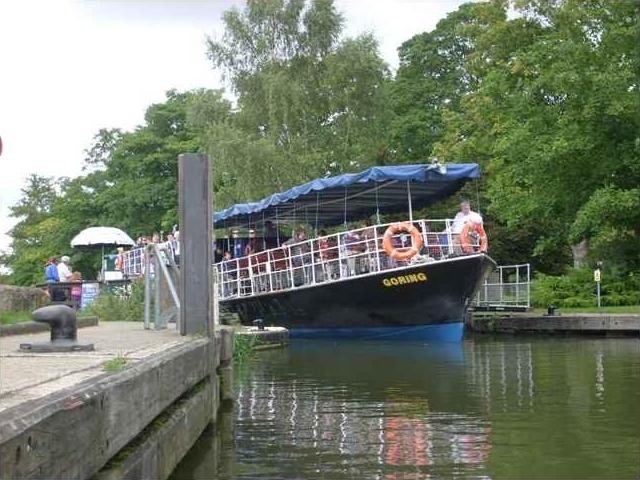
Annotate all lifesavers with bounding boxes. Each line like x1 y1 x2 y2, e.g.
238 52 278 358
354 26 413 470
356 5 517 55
383 222 422 260
116 255 122 269
460 222 487 254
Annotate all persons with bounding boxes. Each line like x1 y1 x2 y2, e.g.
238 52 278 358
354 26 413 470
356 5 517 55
137 231 180 266
215 221 382 280
45 255 81 283
443 200 483 233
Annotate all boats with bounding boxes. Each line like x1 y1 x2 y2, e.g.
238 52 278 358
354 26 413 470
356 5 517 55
121 160 500 344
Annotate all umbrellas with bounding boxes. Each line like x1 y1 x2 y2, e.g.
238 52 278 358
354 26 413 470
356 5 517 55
71 227 135 281
